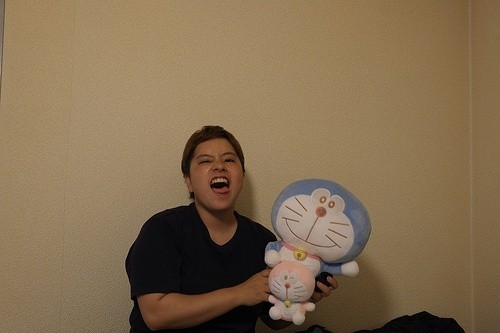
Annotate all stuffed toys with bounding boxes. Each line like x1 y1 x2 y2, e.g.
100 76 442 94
265 177 372 276
267 262 318 325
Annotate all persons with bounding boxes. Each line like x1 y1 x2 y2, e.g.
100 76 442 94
125 121 334 333
